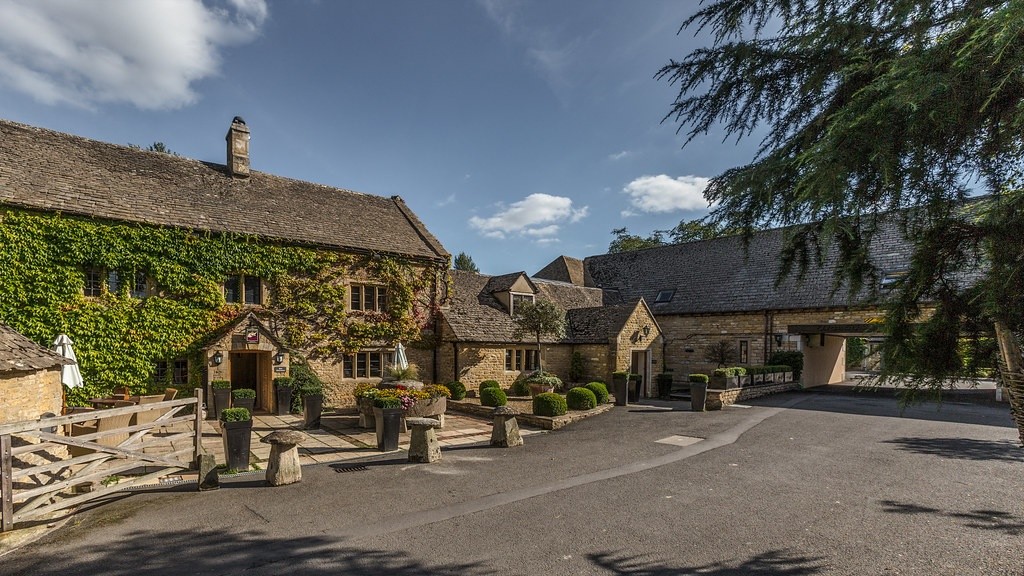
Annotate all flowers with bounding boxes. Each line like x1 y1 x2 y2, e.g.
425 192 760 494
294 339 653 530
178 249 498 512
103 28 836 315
399 393 417 411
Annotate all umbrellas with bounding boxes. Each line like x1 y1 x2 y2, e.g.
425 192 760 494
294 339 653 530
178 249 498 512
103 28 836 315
392 343 409 370
53 334 84 414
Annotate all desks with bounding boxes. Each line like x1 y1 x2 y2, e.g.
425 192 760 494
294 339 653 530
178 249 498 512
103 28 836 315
89 397 118 408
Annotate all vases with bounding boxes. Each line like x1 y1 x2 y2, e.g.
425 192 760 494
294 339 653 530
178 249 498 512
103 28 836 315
398 411 407 435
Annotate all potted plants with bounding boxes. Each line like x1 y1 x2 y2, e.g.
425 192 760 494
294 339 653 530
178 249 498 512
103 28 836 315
517 293 565 399
300 387 323 429
689 374 709 412
657 374 673 401
211 380 232 420
613 371 630 406
711 364 793 391
274 377 294 416
219 407 253 473
628 374 642 402
232 387 256 410
372 397 403 452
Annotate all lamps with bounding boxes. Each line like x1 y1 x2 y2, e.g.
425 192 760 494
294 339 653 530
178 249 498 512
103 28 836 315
275 350 284 364
212 349 223 366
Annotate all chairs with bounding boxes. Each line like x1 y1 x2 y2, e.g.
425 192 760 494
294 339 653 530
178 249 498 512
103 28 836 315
64 388 178 456
